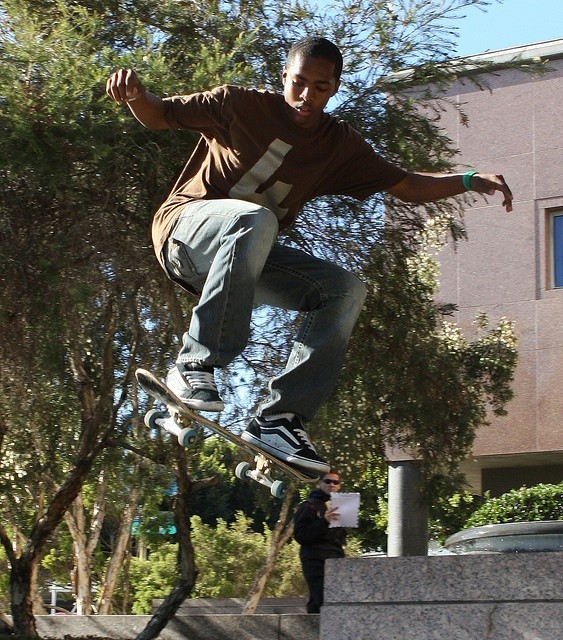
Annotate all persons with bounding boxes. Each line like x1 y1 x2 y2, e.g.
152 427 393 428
105 38 513 474
293 470 346 614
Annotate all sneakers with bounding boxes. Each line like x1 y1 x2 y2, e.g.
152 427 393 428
163 360 225 412
240 413 331 473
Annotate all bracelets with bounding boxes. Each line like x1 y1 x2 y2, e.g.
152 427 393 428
462 171 481 190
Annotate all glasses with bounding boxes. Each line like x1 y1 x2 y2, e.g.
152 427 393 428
322 478 342 486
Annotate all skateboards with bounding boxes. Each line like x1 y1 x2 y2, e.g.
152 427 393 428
135 368 320 497
